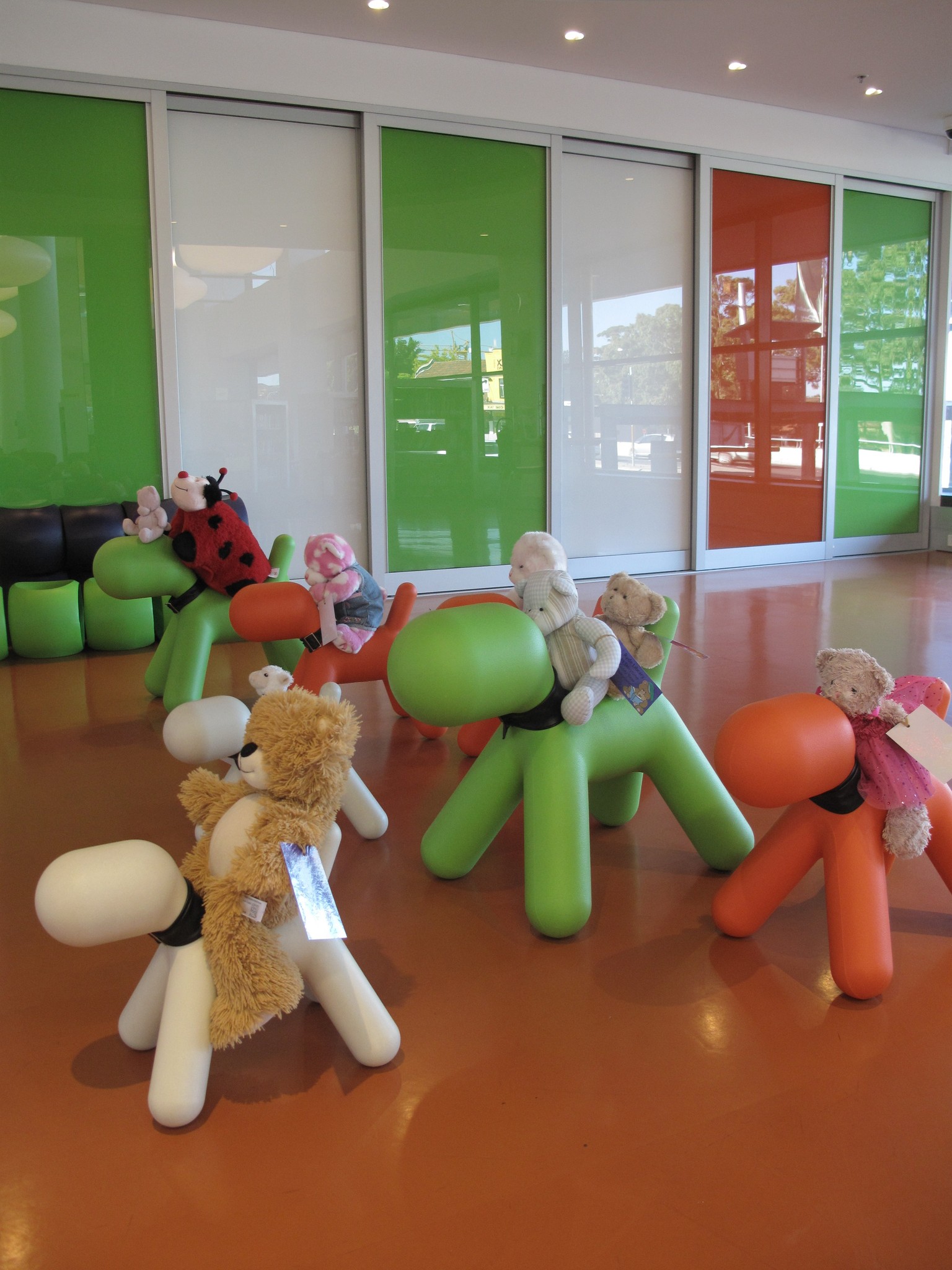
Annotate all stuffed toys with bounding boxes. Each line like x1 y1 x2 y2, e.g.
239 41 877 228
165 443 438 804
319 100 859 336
506 530 566 609
815 645 933 861
516 568 622 727
123 485 168 544
161 468 274 599
304 532 384 655
159 687 358 1051
583 571 668 698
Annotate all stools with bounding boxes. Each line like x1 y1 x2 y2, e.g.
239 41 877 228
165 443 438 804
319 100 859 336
5 579 85 657
81 576 156 650
